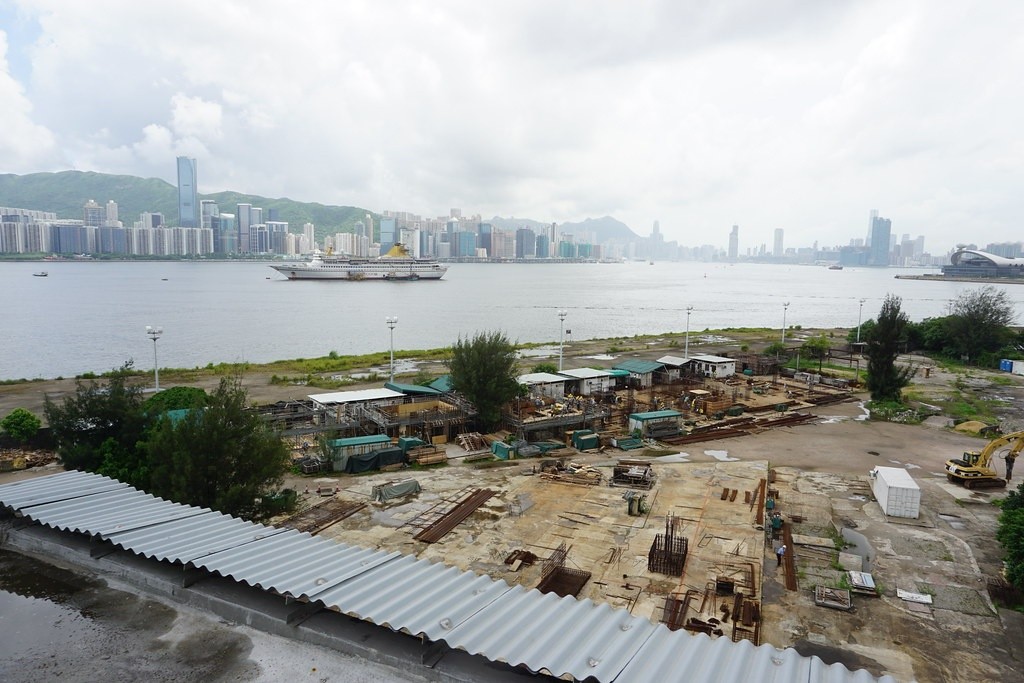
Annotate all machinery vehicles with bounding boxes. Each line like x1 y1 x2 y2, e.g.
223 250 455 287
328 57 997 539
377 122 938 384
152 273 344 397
944 430 1024 489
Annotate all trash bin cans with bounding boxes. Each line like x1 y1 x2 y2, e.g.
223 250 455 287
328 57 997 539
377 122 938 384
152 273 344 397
1000 358 1013 372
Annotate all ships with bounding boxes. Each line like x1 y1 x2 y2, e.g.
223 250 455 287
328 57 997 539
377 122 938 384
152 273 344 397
268 242 448 281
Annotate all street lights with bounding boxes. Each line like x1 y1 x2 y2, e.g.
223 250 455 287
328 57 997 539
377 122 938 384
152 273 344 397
857 299 866 342
684 305 693 360
557 309 568 371
385 316 399 384
781 302 791 343
145 325 163 394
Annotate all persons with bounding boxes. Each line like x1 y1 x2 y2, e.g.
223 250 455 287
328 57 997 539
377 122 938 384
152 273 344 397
535 385 703 429
776 545 787 566
725 371 794 402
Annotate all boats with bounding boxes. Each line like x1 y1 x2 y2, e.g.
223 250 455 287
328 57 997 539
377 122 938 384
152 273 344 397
32 272 47 276
828 265 843 270
